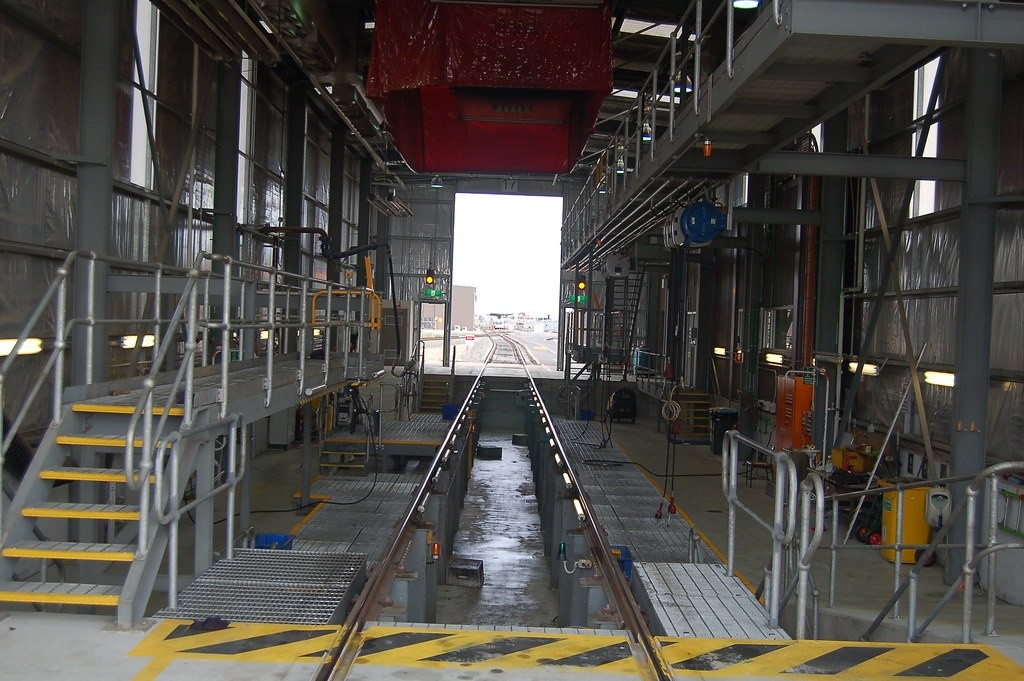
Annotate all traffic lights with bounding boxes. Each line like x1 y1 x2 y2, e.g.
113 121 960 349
579 275 586 290
425 268 435 285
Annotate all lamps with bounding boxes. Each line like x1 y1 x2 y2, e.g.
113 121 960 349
365 188 414 219
599 51 694 192
430 173 444 187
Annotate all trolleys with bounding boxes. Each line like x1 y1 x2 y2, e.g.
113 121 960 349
856 429 931 546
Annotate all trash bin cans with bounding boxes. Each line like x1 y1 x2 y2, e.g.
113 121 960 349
709 408 736 455
878 476 937 567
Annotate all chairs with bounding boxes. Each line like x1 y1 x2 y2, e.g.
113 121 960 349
745 444 774 488
351 387 381 436
